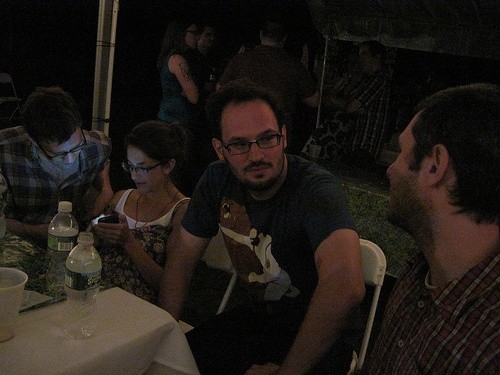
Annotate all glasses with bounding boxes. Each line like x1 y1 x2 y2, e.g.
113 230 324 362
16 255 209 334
34 124 87 160
121 159 162 176
220 127 282 155
186 29 199 36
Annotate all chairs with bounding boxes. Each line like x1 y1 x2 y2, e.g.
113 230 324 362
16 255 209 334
216 238 387 375
0 71 23 122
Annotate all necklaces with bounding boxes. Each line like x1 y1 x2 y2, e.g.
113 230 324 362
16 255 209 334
135 188 179 230
424 267 440 289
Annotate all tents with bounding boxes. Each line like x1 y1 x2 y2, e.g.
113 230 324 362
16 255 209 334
308 0 500 130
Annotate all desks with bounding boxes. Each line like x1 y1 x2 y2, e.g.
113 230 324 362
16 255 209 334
0 229 202 375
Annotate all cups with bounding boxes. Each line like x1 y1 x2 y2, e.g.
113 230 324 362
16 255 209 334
0 266 29 342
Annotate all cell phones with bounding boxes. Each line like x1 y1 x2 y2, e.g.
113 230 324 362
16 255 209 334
98 214 119 224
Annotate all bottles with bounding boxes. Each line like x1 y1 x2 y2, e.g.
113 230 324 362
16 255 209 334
0 167 10 240
64 232 102 339
46 201 79 296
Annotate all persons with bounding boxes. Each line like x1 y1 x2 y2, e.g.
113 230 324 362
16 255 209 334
87 121 192 306
157 18 216 197
215 22 320 154
158 87 365 375
345 39 395 179
364 83 500 375
0 87 113 298
